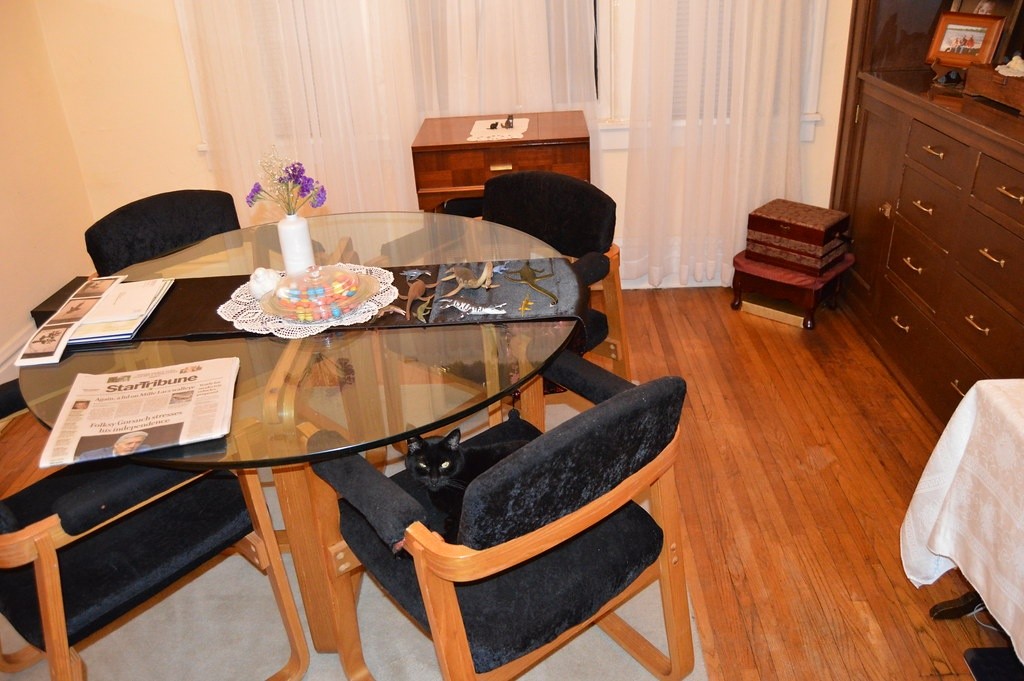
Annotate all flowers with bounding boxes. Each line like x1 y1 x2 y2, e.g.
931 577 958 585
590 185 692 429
244 145 327 214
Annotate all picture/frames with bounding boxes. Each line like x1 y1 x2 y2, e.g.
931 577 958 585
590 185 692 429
923 9 1006 70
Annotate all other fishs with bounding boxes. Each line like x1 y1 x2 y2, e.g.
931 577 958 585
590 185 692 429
398 279 439 321
441 262 493 297
492 261 511 274
412 299 431 323
518 297 534 317
504 260 559 306
370 305 407 323
399 269 432 281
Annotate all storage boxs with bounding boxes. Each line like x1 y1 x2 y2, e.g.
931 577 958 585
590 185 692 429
746 197 851 277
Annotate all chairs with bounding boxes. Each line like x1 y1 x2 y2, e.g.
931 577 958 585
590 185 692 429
432 171 633 408
1 380 309 681
85 188 242 274
292 343 696 681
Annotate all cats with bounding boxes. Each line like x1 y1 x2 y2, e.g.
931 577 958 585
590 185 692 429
501 115 513 129
486 122 498 129
404 424 530 513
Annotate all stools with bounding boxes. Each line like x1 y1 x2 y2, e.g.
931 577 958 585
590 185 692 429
730 248 856 329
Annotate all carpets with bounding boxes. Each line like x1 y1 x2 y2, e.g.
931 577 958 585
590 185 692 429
0 384 673 681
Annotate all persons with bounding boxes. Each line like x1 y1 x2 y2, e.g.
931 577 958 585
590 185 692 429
950 34 975 54
79 431 151 462
973 0 999 15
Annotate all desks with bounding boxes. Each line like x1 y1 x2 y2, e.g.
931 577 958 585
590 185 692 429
18 209 585 681
898 376 1024 681
410 110 592 216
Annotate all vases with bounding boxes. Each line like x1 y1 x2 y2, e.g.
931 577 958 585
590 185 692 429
276 212 315 273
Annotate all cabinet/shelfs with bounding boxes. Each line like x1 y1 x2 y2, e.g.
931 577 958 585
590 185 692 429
821 0 1024 432
